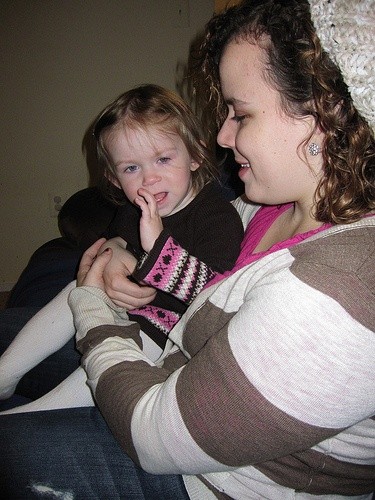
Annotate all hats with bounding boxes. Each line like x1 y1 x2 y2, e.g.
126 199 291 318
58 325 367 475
309 1 375 131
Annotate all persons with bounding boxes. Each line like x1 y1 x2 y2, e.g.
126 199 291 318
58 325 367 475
0 0 375 500
1 83 244 414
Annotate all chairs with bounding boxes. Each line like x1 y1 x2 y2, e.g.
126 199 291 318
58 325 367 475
7 150 244 307
